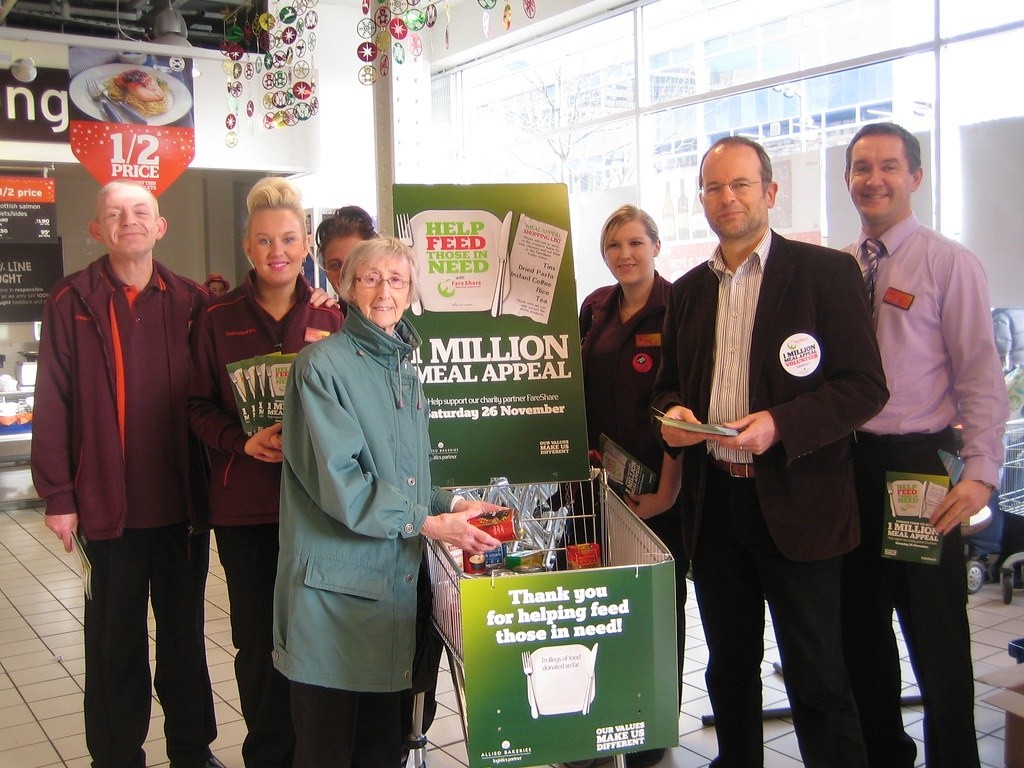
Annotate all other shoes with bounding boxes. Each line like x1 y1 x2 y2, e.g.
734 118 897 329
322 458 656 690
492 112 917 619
625 749 664 767
170 758 222 768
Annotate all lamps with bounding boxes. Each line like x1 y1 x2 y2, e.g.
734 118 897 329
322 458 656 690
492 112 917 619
150 6 196 48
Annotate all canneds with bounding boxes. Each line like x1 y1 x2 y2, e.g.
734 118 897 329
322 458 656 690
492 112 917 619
463 550 487 575
467 507 523 542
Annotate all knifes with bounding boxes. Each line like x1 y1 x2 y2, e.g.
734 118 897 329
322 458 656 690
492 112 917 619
581 642 598 716
490 210 513 317
918 480 927 518
97 80 147 125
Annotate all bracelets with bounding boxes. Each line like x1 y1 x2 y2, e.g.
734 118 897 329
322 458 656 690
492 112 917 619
981 480 991 487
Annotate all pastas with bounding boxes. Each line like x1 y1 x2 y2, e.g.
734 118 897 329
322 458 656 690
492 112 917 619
108 79 170 116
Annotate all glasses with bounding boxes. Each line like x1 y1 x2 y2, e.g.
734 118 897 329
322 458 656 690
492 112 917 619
700 179 763 201
353 274 411 289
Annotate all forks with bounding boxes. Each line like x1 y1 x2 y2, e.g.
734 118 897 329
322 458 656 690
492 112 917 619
266 365 275 399
396 214 422 316
85 79 123 124
887 481 896 518
521 650 538 719
229 372 247 403
256 365 265 397
243 368 256 400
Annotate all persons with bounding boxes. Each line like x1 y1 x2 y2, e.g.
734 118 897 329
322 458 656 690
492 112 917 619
645 136 890 768
30 178 340 768
571 206 691 768
272 236 507 768
191 176 343 768
316 206 443 735
841 124 1010 768
204 274 229 296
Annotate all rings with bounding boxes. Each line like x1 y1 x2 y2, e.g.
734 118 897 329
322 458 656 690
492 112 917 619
740 446 742 450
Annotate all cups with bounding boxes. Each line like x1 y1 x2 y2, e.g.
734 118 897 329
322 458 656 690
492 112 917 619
151 54 177 72
117 50 147 65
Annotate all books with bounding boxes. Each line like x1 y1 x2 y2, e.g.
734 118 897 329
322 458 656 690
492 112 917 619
71 532 92 599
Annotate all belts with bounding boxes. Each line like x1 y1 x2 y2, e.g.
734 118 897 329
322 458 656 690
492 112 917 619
710 460 756 478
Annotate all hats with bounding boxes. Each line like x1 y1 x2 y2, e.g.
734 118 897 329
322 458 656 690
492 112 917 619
204 274 230 292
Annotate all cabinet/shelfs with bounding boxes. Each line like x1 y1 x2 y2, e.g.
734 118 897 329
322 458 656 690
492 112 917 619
0 392 48 510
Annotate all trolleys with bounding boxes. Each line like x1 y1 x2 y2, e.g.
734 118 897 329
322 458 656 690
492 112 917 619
998 418 1024 603
398 445 686 768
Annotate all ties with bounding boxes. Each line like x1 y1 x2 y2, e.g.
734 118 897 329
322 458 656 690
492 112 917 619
861 239 885 326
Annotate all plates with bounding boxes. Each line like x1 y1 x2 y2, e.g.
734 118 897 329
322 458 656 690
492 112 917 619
248 366 256 392
529 643 595 716
260 363 266 388
69 63 193 126
409 210 511 313
893 479 927 517
234 368 246 399
271 363 293 397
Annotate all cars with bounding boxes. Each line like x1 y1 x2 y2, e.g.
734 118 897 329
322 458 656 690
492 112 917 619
989 305 1023 375
